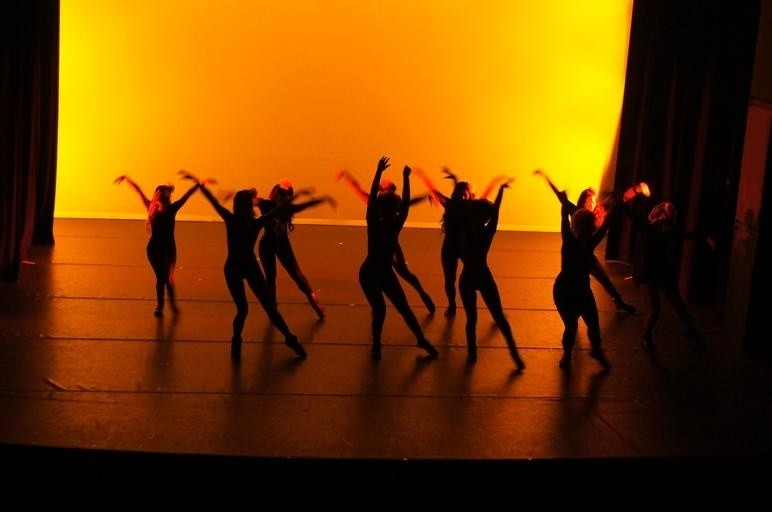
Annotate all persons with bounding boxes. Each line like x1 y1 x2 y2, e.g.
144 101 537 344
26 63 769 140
115 175 217 318
532 168 718 371
335 154 526 372
178 168 338 372
